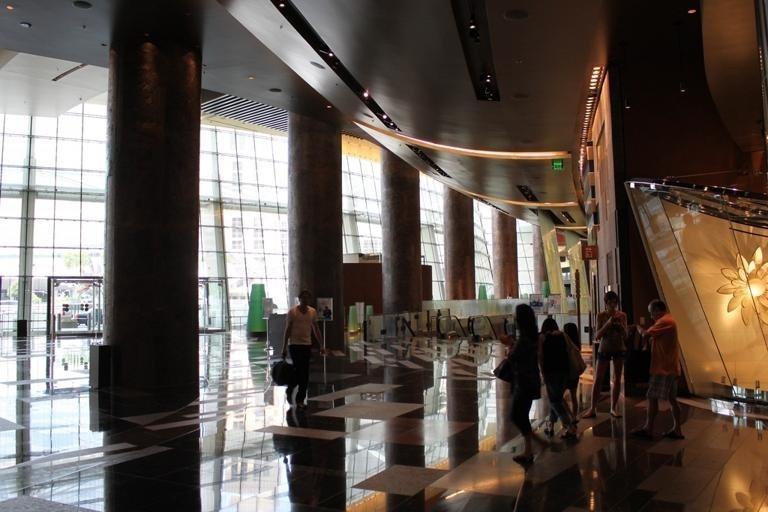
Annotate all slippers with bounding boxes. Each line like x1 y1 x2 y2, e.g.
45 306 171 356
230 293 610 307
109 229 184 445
629 425 659 440
662 429 685 439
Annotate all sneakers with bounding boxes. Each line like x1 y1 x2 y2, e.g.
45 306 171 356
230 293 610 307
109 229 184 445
513 454 533 464
583 409 596 418
286 388 293 404
296 399 306 408
611 409 623 418
545 428 554 435
565 431 578 445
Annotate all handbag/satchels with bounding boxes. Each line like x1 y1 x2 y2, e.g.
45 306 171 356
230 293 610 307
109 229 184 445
272 361 294 384
568 344 586 374
493 359 512 383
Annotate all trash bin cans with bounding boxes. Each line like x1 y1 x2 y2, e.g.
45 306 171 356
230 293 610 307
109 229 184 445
89 344 110 392
268 313 288 347
17 320 27 337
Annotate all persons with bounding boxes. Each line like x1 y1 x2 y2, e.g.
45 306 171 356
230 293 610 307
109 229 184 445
513 463 534 512
537 319 580 440
281 290 326 408
581 291 628 418
499 304 548 465
323 306 331 320
283 408 320 506
564 323 581 425
567 294 577 315
629 299 683 439
